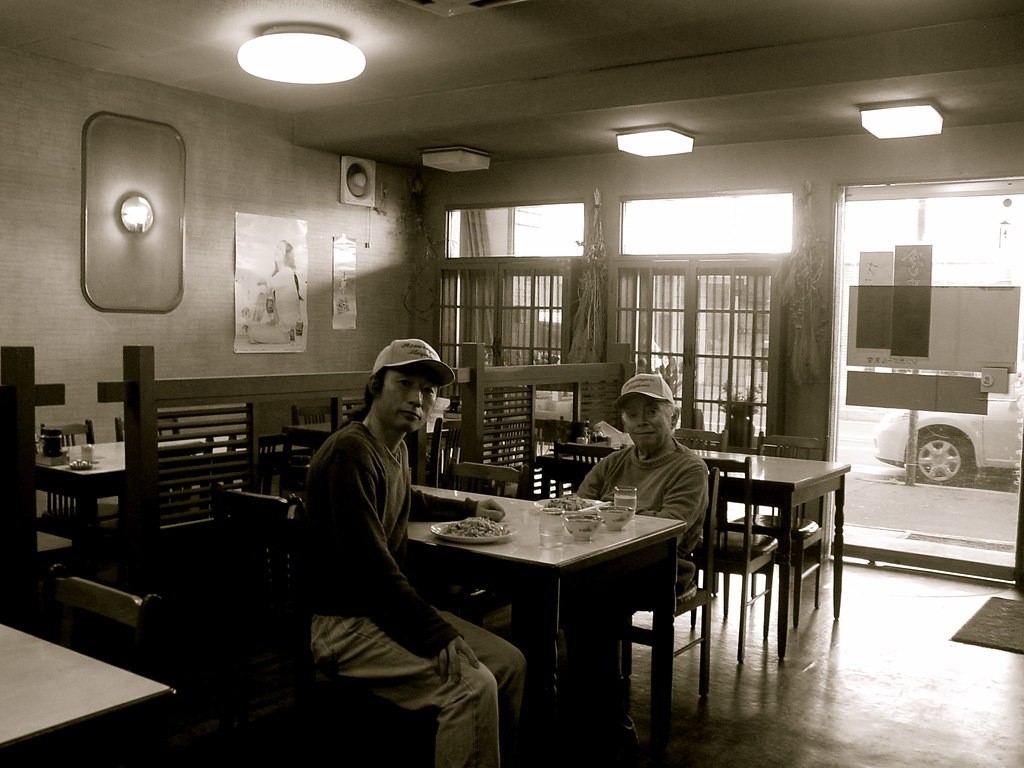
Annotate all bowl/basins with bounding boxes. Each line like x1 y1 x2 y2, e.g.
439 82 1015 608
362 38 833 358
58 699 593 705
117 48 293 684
596 506 635 531
564 514 604 544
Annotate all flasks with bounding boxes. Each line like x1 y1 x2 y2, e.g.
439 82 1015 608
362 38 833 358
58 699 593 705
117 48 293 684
40 428 64 458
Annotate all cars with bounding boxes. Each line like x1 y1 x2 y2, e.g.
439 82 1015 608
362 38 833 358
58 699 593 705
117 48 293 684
871 373 1024 487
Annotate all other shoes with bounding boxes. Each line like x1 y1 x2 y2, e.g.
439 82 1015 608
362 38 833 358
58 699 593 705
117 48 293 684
620 716 640 747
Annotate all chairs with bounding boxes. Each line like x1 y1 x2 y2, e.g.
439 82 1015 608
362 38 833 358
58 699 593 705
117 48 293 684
35 397 833 768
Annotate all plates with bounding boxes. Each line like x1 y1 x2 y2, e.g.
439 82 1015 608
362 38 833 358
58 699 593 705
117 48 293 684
533 497 610 515
429 520 520 544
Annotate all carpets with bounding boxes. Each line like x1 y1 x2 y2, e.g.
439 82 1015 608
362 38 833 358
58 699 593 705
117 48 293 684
951 596 1024 654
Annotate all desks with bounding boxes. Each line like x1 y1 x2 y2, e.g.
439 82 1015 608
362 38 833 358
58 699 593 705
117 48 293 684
406 486 687 763
281 423 455 486
35 436 309 537
560 447 851 659
0 625 176 768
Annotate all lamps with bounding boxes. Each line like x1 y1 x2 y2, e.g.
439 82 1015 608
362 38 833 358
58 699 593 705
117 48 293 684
236 25 367 86
420 148 490 174
614 123 694 156
858 102 945 141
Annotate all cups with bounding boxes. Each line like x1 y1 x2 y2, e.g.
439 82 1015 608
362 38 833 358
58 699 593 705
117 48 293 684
538 508 564 548
589 432 597 446
613 485 637 519
81 444 95 464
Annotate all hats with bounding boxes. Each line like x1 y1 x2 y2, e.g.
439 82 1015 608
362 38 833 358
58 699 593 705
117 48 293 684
613 372 673 413
372 339 455 386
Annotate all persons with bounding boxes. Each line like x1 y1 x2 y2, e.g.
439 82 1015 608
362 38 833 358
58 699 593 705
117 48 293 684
308 339 526 768
247 240 302 344
561 373 709 756
